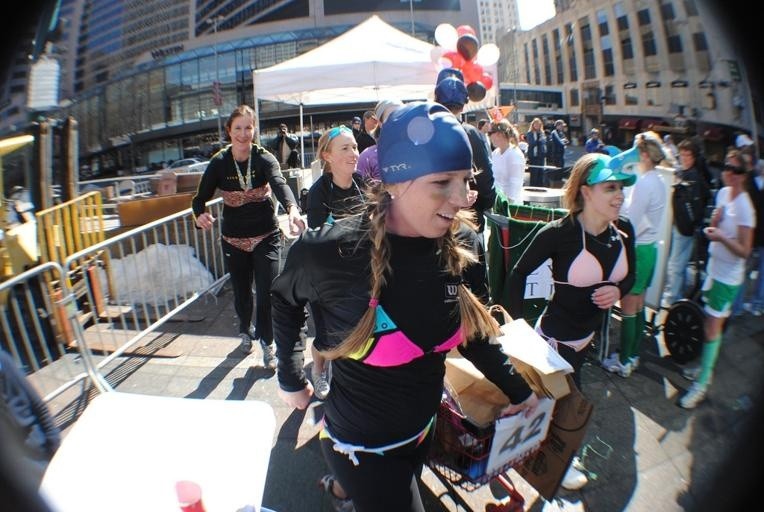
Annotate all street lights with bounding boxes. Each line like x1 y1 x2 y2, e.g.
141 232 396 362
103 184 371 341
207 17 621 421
205 15 226 149
507 26 520 124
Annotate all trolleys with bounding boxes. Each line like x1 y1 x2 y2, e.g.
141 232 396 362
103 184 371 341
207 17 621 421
427 389 558 511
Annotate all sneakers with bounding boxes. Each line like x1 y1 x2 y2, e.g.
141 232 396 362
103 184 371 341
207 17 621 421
321 473 354 512
602 359 633 377
259 339 278 368
681 366 714 382
610 352 642 371
310 366 331 399
237 321 254 354
680 383 709 409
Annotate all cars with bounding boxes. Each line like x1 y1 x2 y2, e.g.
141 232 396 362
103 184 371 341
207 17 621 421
157 157 210 173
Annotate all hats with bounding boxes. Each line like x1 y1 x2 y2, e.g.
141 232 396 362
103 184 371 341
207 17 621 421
435 77 468 106
373 98 403 121
377 100 474 185
585 158 636 188
352 116 361 123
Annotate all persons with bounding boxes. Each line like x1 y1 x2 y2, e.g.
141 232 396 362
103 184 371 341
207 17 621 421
477 116 491 148
682 151 758 410
266 102 539 511
191 104 306 371
549 120 569 169
603 133 670 382
432 69 496 226
271 123 300 169
358 110 378 149
351 117 361 133
735 134 764 204
506 154 637 395
357 97 406 181
653 140 706 307
584 127 605 152
724 151 745 168
525 119 545 187
307 125 374 227
678 119 703 150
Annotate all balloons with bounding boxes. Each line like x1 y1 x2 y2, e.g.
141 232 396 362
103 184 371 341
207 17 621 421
456 25 475 38
468 82 485 102
477 43 500 67
455 35 479 62
432 23 459 50
462 63 483 82
441 52 460 68
480 73 493 88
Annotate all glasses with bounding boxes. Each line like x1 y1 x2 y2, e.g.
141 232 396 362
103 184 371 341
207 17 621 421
723 163 745 175
322 125 353 152
